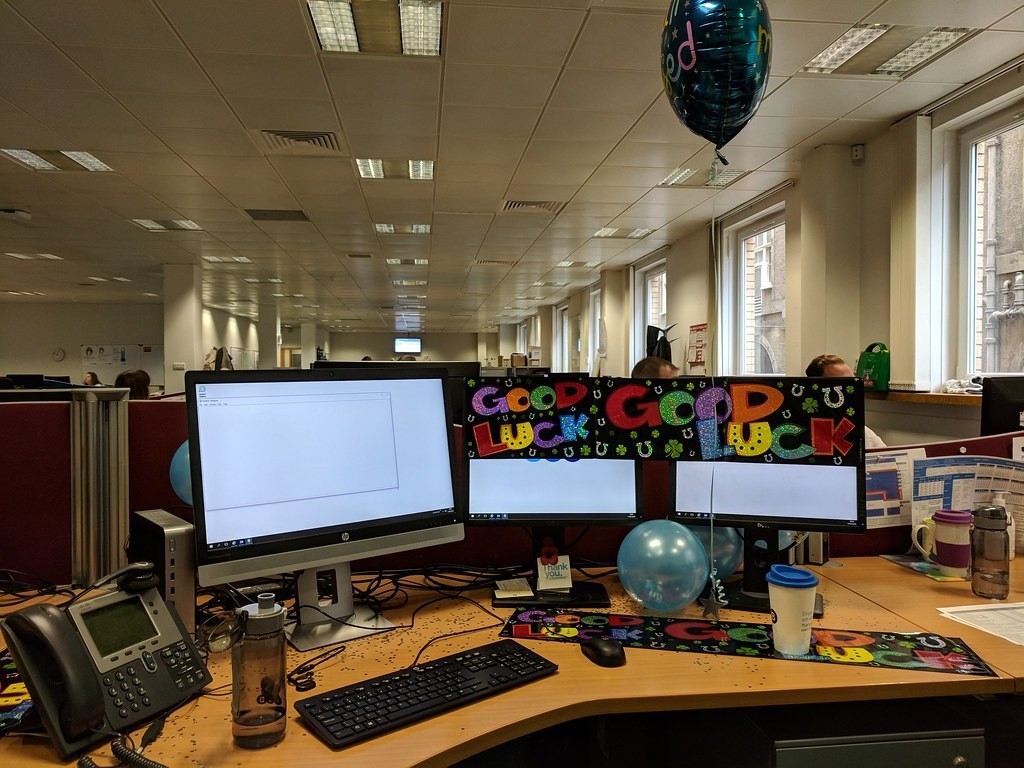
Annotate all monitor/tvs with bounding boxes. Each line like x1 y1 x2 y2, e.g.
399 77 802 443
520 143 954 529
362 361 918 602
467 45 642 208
394 337 422 355
980 376 1024 437
185 361 865 617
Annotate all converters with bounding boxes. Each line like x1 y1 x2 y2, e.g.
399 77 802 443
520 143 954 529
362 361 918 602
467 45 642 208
217 582 283 610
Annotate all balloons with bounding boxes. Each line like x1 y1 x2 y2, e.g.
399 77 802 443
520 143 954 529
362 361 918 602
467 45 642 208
657 0 773 166
170 439 192 506
618 517 798 613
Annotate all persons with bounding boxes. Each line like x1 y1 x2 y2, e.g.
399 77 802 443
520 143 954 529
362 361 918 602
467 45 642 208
84 372 102 386
805 354 889 448
114 370 151 400
396 355 417 361
361 356 373 361
632 357 680 378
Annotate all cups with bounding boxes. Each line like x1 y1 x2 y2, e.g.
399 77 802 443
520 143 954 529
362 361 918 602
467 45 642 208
912 518 939 564
932 509 972 578
766 564 820 654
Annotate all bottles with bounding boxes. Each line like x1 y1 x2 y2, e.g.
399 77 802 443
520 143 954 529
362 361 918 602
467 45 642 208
971 506 1012 600
198 593 288 749
121 348 125 362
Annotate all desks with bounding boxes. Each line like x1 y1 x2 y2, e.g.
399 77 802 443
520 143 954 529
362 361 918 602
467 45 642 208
0 553 1024 767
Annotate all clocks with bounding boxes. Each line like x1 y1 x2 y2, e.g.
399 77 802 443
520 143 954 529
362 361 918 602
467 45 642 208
51 347 65 361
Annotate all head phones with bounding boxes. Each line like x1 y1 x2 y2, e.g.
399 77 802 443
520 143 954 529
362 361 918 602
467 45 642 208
59 561 159 616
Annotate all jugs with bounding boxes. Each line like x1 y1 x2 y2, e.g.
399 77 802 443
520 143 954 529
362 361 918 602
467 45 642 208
86 347 92 356
99 347 104 354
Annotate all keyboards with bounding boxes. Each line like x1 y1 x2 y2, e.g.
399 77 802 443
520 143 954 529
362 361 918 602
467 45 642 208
294 639 558 750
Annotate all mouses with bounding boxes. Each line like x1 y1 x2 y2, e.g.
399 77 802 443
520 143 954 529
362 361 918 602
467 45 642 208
580 637 626 668
